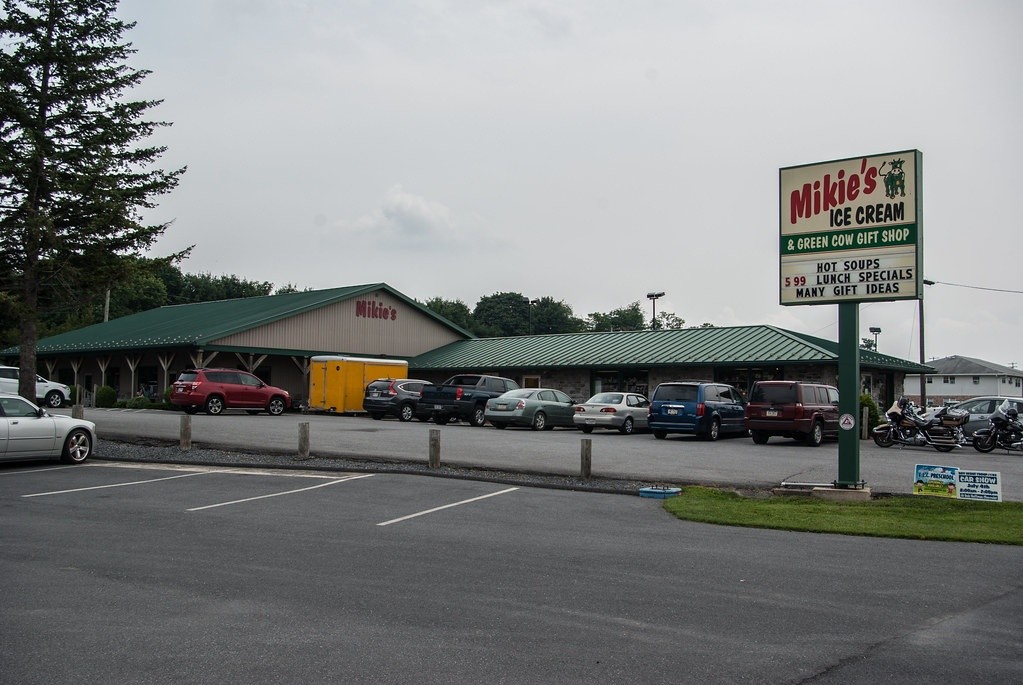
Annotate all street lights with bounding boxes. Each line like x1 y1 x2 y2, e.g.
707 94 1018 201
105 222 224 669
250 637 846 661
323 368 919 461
646 292 665 330
869 327 881 353
523 298 537 335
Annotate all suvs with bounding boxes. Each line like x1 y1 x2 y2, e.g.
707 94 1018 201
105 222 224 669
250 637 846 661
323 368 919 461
170 367 293 416
362 377 437 423
744 379 839 447
0 365 72 408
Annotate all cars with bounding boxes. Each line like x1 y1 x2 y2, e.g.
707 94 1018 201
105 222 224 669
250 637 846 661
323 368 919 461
0 395 98 465
573 391 651 434
928 395 1023 442
483 388 581 432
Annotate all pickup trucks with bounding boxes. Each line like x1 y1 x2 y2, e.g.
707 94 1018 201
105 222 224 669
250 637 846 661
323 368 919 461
416 372 522 426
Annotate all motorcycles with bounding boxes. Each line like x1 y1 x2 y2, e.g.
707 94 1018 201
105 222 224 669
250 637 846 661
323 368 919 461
872 396 970 452
972 399 1023 452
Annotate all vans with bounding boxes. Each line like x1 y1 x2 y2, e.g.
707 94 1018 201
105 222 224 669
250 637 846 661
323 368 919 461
647 379 752 442
